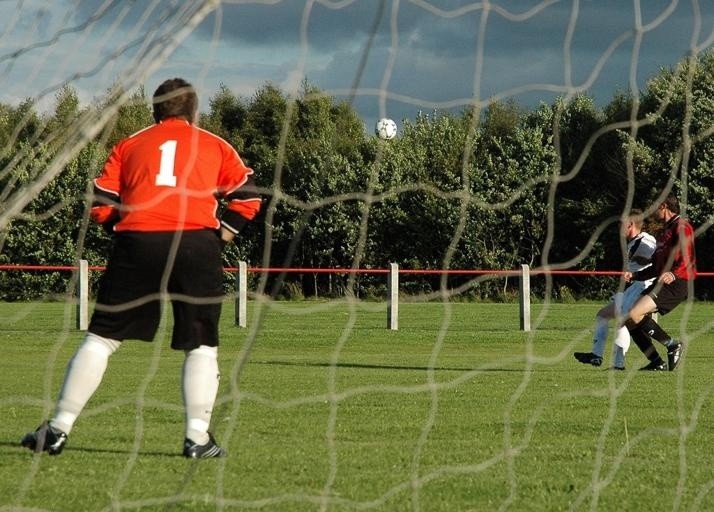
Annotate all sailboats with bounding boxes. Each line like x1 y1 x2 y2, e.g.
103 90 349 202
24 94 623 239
375 118 397 139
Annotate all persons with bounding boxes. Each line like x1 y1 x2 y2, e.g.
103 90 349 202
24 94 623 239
622 191 699 371
19 78 264 460
573 208 657 371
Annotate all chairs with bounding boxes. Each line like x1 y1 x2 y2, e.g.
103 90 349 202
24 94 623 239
184 432 227 457
638 360 666 371
667 339 684 371
574 351 603 366
614 361 625 370
20 420 68 456
